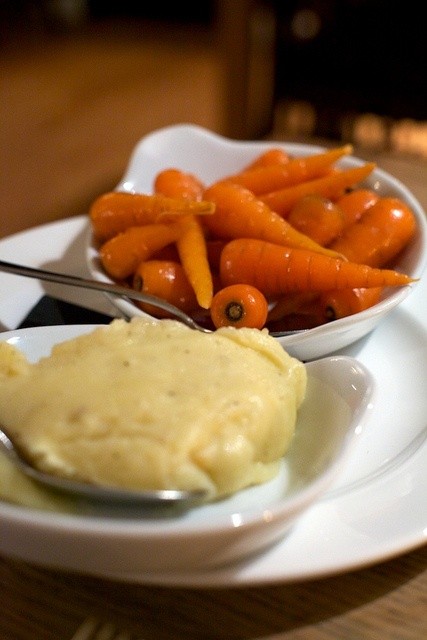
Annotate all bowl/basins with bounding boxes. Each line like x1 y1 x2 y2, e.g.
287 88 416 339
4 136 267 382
87 123 427 359
1 324 375 572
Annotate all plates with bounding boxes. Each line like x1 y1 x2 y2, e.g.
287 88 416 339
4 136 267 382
0 210 426 593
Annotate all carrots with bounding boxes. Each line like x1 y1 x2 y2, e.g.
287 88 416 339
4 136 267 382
89 144 421 331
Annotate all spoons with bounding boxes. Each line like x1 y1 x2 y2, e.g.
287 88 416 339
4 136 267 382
1 427 207 520
0 258 312 334
